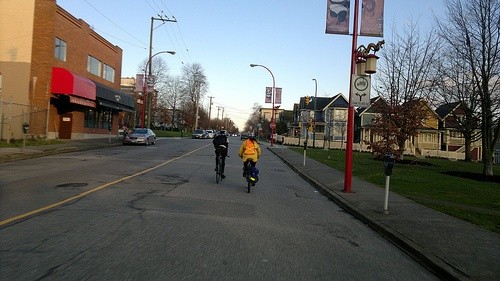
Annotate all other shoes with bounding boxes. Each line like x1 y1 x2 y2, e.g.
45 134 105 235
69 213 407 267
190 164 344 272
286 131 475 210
214 168 219 171
219 173 225 178
243 172 246 178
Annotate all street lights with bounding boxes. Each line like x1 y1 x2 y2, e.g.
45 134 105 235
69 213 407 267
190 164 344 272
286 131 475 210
312 78 317 149
141 50 176 128
251 64 275 147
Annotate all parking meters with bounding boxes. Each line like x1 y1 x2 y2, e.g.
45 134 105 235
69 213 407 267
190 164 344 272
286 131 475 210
382 154 393 211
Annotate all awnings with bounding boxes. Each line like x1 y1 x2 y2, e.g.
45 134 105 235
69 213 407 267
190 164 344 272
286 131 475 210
50 67 135 114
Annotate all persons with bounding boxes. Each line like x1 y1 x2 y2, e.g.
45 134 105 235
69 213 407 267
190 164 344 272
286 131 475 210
212 131 230 179
239 131 261 178
123 126 132 139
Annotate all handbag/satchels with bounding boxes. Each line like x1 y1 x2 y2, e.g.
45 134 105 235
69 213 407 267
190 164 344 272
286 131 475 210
249 167 259 187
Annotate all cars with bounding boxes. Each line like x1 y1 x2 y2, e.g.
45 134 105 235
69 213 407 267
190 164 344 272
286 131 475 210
192 129 207 139
123 127 156 146
205 129 251 140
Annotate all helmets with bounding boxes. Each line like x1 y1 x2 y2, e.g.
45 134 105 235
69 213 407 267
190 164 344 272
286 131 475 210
248 132 254 137
219 129 226 136
